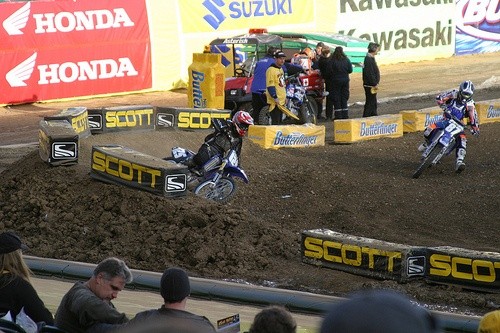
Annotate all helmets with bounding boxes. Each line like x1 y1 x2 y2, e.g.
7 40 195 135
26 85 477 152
459 80 475 104
302 47 313 58
232 111 254 137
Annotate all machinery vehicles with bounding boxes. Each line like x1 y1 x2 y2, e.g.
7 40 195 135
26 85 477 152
225 28 324 122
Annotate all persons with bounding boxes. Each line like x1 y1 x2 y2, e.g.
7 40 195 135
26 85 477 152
251 47 306 125
321 290 437 333
418 80 480 174
363 43 380 118
90 267 217 333
189 110 254 176
54 258 132 333
249 307 297 333
291 48 314 73
311 42 325 120
318 47 333 119
327 47 353 120
266 51 286 125
0 231 54 333
477 310 500 333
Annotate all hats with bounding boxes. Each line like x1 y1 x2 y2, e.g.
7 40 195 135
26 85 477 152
0 234 22 252
273 51 287 58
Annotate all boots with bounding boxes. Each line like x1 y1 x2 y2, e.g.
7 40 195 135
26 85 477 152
342 109 349 119
331 109 342 122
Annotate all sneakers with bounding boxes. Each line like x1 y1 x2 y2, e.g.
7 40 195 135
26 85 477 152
418 142 428 151
454 159 465 173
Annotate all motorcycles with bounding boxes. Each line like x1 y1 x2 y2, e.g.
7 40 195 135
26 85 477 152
163 128 249 203
411 100 480 178
259 69 318 124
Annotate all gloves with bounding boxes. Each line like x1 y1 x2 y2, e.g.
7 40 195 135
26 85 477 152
223 126 234 140
470 123 480 135
441 103 452 114
